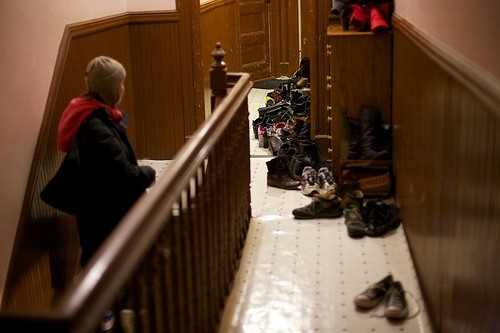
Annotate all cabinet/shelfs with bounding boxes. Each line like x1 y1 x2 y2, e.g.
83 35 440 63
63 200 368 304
326 22 391 186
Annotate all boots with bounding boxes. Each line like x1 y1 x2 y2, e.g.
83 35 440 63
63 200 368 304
342 106 390 161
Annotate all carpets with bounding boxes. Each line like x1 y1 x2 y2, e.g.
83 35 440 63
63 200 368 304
252 79 289 89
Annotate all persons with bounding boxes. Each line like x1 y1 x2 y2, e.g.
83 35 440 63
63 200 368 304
293 57 310 79
39 56 155 269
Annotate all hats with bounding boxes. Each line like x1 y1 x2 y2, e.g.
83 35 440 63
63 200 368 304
84 56 126 107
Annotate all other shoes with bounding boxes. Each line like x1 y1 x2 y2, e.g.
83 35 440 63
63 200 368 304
383 280 408 318
363 199 402 236
317 167 338 194
343 190 366 237
292 192 343 219
251 74 318 189
99 310 116 331
353 274 395 308
300 166 320 196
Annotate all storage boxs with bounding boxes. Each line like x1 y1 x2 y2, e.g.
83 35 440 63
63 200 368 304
343 170 389 194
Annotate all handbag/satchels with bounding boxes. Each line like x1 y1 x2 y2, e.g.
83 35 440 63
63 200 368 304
40 146 107 218
